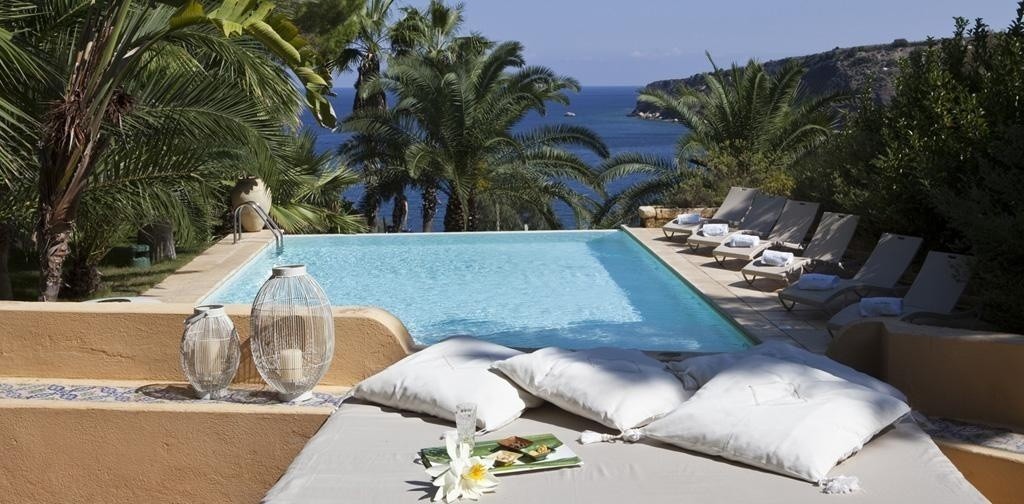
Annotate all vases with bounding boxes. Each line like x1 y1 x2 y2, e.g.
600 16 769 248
231 175 273 231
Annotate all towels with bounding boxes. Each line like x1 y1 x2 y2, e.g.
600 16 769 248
677 212 699 225
727 235 759 248
796 272 842 289
858 296 904 314
702 223 730 236
761 248 794 266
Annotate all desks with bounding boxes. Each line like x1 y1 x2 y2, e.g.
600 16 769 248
261 382 995 502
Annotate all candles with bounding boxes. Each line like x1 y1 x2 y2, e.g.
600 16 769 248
200 339 221 374
280 349 302 380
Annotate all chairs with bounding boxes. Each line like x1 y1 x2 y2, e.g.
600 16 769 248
690 193 784 258
823 249 979 337
661 186 756 243
779 232 922 314
713 198 819 268
745 211 860 290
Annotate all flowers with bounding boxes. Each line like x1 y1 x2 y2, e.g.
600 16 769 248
430 440 504 504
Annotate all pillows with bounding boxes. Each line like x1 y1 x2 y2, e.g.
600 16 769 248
505 338 687 441
666 345 915 406
627 353 903 482
355 328 547 428
